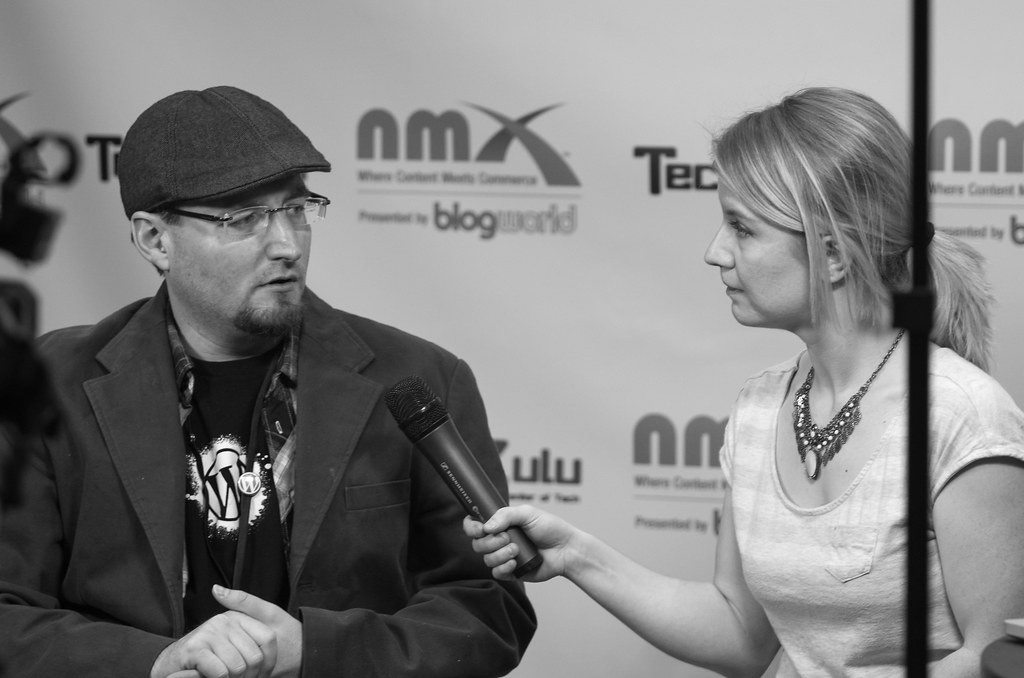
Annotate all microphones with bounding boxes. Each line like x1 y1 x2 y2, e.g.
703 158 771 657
383 376 543 579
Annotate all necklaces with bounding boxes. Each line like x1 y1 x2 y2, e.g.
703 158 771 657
791 325 908 480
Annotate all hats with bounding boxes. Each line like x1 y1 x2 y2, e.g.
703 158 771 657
117 86 332 221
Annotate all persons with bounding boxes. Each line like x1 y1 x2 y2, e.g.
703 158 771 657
0 86 538 678
463 88 1024 678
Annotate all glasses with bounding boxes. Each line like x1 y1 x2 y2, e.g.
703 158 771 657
165 190 331 236
